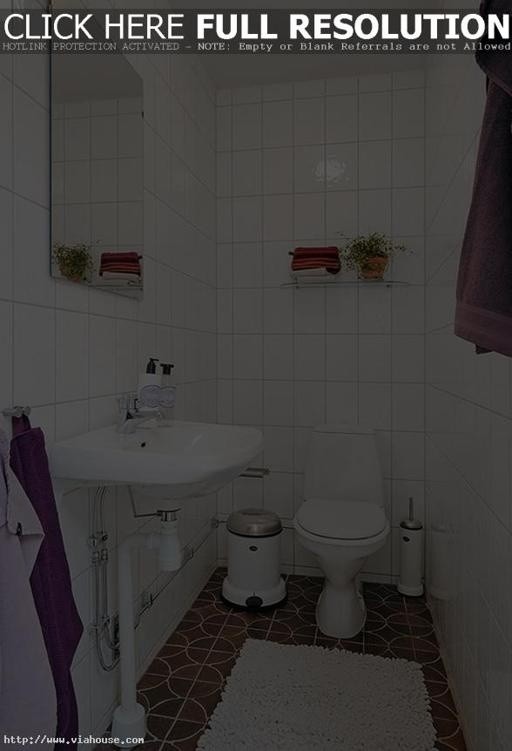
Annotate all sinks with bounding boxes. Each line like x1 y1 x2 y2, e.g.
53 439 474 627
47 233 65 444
52 420 263 499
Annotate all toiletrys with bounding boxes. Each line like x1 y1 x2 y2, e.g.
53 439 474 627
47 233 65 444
159 362 175 422
142 358 160 412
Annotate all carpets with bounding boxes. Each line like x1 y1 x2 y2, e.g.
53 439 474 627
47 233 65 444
196 638 439 751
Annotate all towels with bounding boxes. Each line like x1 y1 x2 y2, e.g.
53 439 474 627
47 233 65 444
10 405 84 750
0 427 59 750
289 246 341 284
95 251 143 287
453 0 510 360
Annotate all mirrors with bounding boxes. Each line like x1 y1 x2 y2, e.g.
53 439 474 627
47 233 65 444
50 0 144 301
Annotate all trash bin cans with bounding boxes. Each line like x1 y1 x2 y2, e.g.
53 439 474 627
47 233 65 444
222 508 288 610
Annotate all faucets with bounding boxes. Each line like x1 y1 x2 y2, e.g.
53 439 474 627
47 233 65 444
118 392 162 434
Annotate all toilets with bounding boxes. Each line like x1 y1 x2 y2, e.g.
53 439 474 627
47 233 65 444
294 425 391 640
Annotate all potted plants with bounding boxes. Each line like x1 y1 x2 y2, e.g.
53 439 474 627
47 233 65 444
51 239 103 284
337 231 417 281
